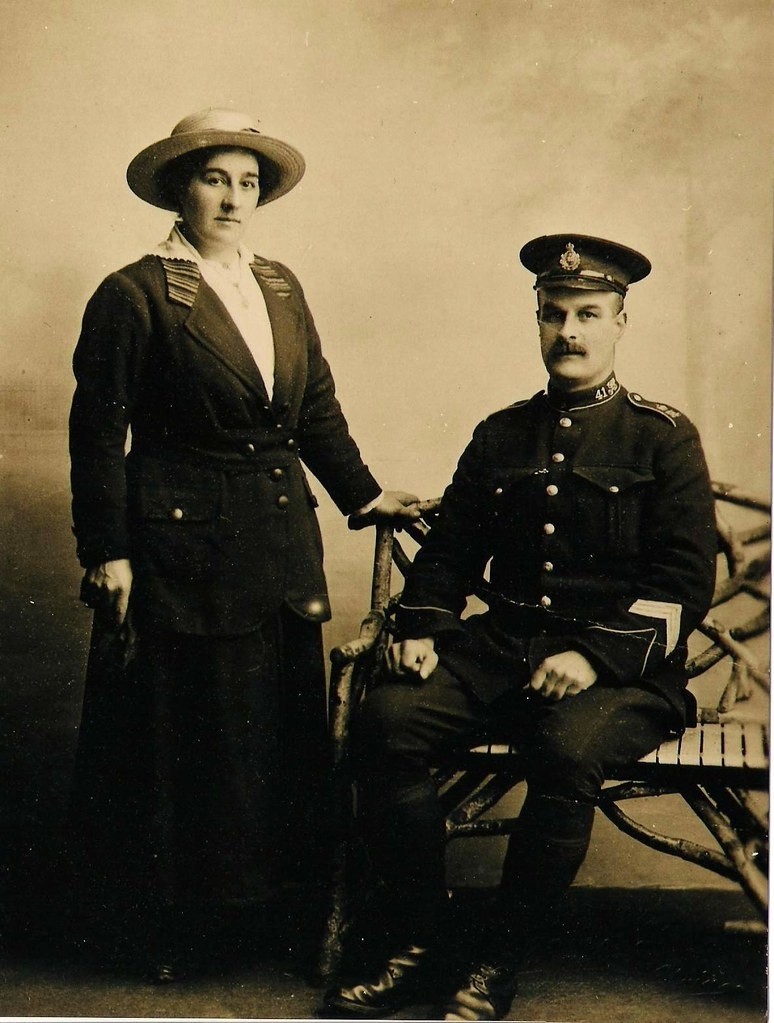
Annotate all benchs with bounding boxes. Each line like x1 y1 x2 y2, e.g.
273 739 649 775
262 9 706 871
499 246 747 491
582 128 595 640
310 482 774 1023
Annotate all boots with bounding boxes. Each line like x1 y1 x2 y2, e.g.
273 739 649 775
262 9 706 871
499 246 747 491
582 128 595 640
326 778 440 1014
433 789 594 1021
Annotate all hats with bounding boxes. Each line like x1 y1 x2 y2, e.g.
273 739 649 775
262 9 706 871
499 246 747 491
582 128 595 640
126 107 306 212
519 233 653 298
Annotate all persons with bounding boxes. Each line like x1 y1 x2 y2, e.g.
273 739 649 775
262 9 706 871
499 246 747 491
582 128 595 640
323 235 719 1021
69 108 421 984
